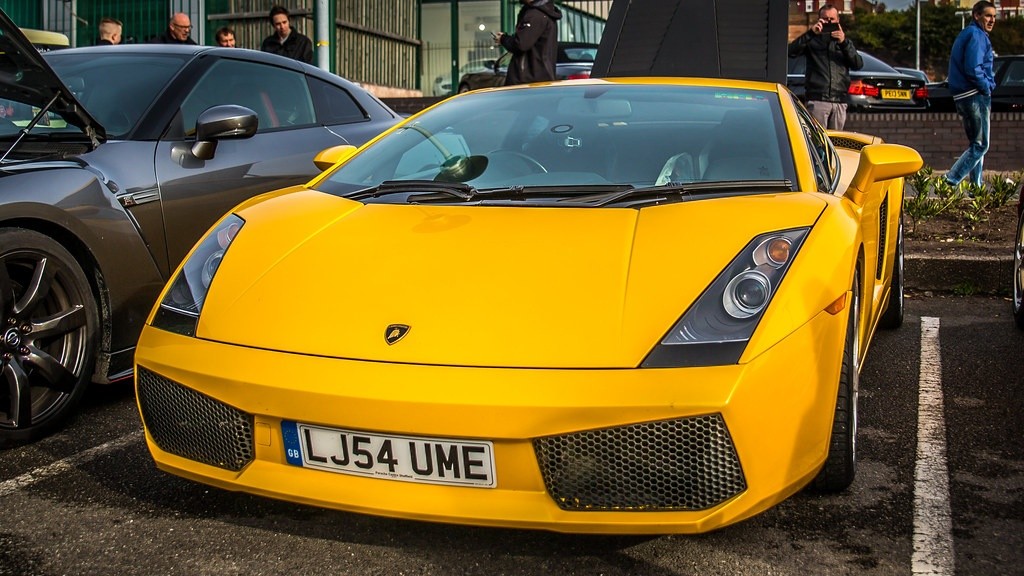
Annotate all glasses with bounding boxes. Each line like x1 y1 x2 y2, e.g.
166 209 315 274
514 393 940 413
173 23 192 30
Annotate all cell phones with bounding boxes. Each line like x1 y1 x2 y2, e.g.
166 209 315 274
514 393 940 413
822 23 840 33
491 31 498 38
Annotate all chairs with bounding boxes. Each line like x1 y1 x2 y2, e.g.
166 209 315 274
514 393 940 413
705 109 784 179
520 115 617 183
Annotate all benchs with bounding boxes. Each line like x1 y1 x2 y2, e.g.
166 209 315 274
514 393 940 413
599 123 715 180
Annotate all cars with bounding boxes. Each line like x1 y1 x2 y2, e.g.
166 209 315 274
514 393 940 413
458 42 1024 114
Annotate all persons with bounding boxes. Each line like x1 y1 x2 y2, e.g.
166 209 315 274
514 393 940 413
215 27 241 48
492 0 563 85
940 0 997 195
147 13 198 45
96 18 123 45
260 5 314 65
787 4 864 131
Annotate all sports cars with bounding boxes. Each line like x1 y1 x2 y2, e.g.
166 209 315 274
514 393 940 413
432 58 498 97
0 7 405 448
134 77 923 534
